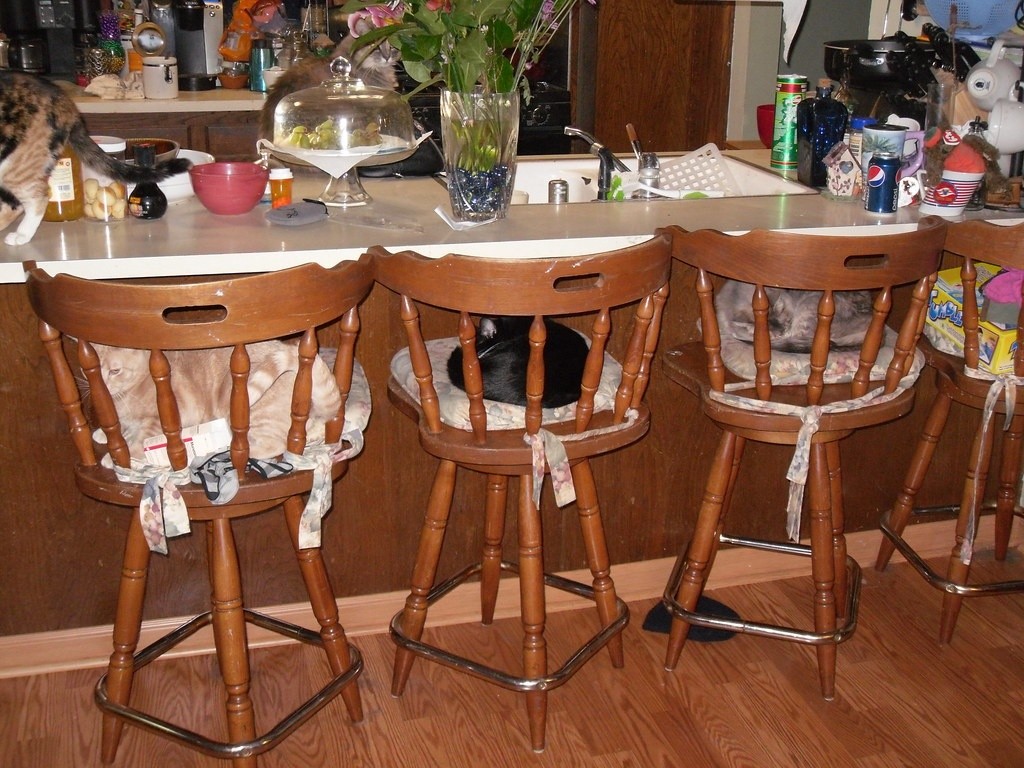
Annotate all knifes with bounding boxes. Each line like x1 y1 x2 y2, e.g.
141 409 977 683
886 23 981 101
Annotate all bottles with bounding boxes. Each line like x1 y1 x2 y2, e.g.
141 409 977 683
250 39 271 92
39 135 167 221
968 115 990 209
277 20 335 69
96 10 125 74
269 168 294 209
797 80 845 187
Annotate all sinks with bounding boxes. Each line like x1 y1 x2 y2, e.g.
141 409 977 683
433 151 822 206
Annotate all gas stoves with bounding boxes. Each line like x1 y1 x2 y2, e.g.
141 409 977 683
382 59 572 136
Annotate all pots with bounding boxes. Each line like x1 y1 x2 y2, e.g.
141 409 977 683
822 39 964 93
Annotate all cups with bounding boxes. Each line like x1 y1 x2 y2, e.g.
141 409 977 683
262 70 285 92
862 124 925 199
143 57 178 99
965 40 1024 154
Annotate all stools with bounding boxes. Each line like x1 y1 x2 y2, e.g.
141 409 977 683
20 253 370 768
657 214 950 698
877 219 1024 642
370 229 672 752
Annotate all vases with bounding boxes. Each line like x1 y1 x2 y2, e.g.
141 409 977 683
441 89 520 222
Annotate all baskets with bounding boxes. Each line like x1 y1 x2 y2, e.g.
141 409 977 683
659 142 743 198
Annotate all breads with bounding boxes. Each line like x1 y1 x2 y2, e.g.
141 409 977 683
82 178 128 220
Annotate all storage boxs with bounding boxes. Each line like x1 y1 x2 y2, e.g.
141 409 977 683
924 262 1024 347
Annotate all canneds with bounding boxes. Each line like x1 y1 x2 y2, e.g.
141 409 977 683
770 74 807 170
864 152 902 213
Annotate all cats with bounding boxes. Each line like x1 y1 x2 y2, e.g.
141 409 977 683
446 315 589 408
68 335 343 469
715 276 886 355
0 70 192 245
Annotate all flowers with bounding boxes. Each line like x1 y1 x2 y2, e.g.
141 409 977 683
349 0 590 96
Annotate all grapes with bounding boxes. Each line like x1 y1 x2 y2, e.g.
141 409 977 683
289 118 383 150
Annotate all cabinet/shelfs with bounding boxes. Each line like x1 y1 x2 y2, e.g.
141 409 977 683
68 112 273 164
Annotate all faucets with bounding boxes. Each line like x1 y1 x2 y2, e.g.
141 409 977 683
564 124 664 202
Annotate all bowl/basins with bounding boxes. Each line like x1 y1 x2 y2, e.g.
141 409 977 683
127 149 214 203
918 168 985 216
124 138 179 166
758 104 773 149
188 161 269 214
217 73 249 87
